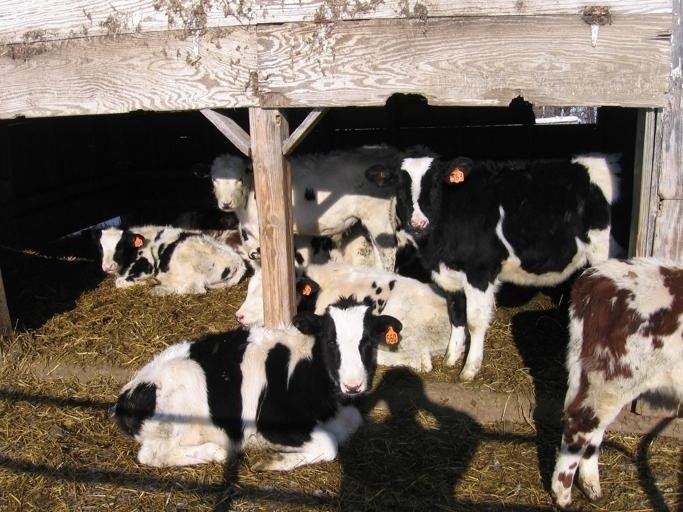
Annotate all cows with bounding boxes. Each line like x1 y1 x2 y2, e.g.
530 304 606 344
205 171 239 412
338 212 421 271
363 141 632 382
234 247 453 374
110 292 404 472
81 223 247 298
549 256 683 512
190 143 406 272
